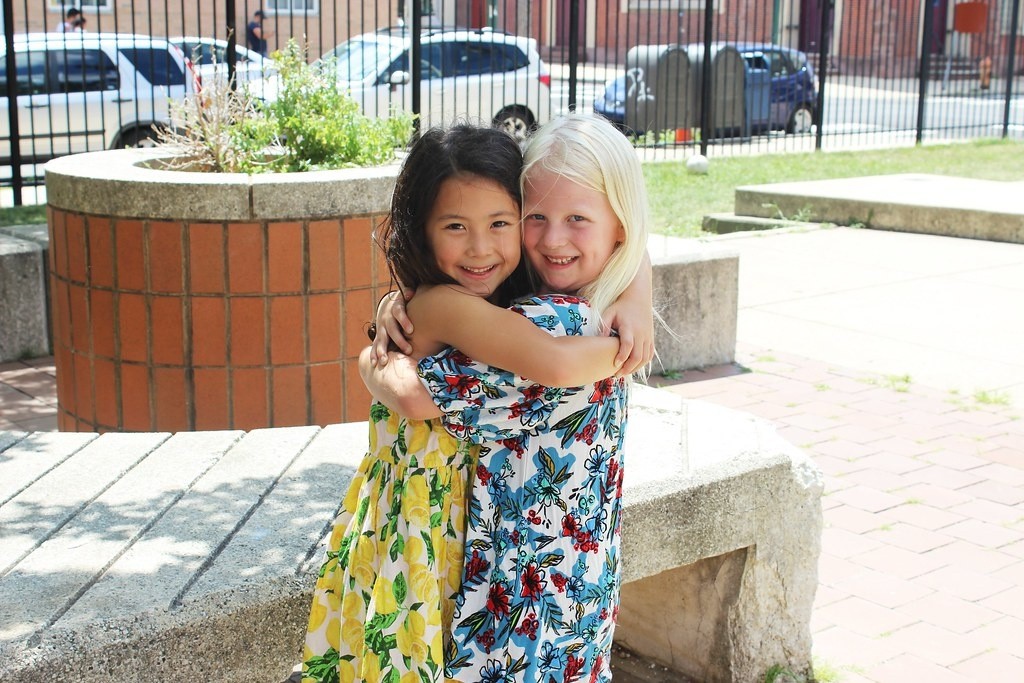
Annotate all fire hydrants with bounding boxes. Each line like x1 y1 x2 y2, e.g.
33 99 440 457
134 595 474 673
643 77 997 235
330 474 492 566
978 56 994 90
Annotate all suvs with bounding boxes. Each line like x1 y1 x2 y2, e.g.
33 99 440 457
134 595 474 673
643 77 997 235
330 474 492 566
232 23 552 150
0 32 204 167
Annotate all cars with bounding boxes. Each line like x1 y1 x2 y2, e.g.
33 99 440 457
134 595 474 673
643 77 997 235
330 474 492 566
592 40 820 142
168 34 283 92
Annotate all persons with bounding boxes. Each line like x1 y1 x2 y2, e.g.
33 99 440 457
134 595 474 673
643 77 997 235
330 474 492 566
301 123 656 683
248 11 273 58
359 114 650 683
57 8 87 32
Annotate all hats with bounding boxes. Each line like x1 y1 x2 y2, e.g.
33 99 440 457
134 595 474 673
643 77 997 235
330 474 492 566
254 11 267 19
